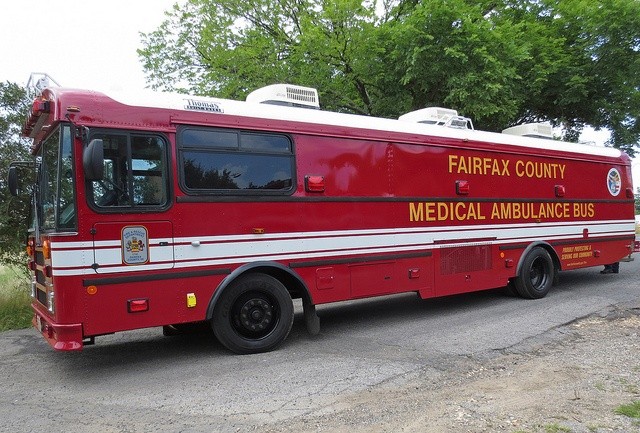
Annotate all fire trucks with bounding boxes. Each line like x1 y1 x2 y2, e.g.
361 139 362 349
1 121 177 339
7 83 637 355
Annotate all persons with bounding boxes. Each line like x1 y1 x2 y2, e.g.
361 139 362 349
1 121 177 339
601 262 620 274
